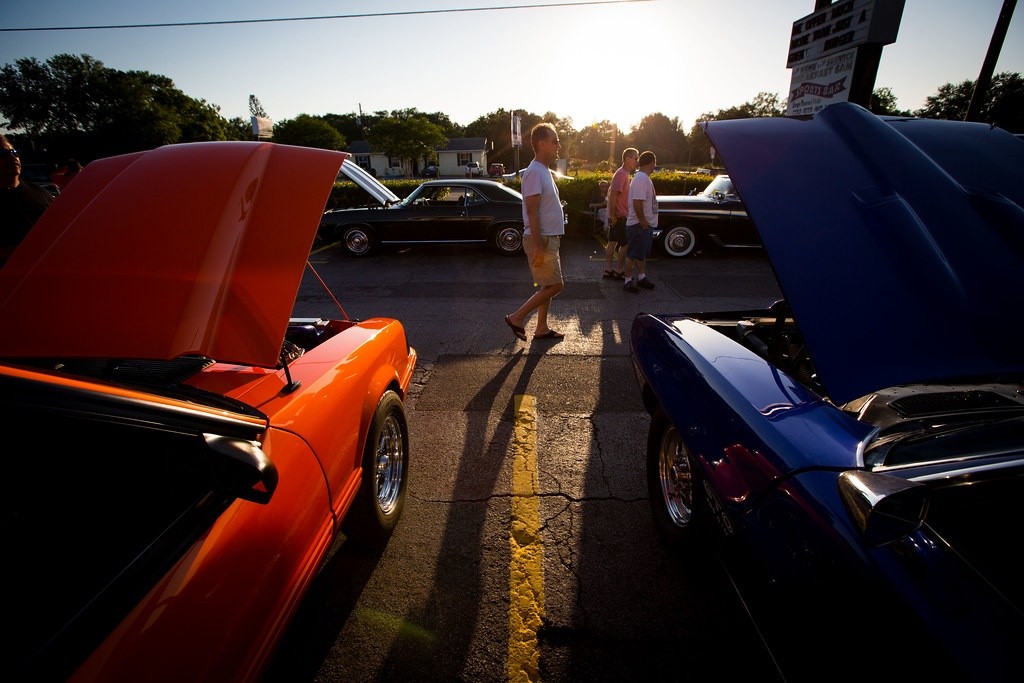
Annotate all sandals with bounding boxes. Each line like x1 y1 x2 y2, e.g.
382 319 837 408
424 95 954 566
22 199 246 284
603 268 625 280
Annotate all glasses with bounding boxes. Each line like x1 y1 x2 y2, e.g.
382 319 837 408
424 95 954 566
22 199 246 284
601 185 608 188
544 137 560 144
0 148 20 158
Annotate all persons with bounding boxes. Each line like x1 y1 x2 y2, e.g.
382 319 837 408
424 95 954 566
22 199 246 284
589 180 610 241
505 122 565 342
0 133 52 269
603 147 640 280
623 151 659 292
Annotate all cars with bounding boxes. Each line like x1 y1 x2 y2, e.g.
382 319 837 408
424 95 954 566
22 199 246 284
639 175 766 258
0 144 419 683
422 166 440 178
631 104 1024 683
317 156 567 256
502 169 527 181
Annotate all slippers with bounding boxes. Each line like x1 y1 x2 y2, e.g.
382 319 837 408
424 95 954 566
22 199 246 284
504 314 527 342
534 329 566 339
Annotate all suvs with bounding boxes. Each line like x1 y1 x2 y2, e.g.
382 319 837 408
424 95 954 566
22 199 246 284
465 161 483 176
490 163 506 177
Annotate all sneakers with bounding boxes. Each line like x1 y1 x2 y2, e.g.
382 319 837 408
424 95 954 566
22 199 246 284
636 277 655 289
623 281 641 293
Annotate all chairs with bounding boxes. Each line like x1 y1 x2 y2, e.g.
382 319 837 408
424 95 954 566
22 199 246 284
583 203 605 238
458 195 463 206
